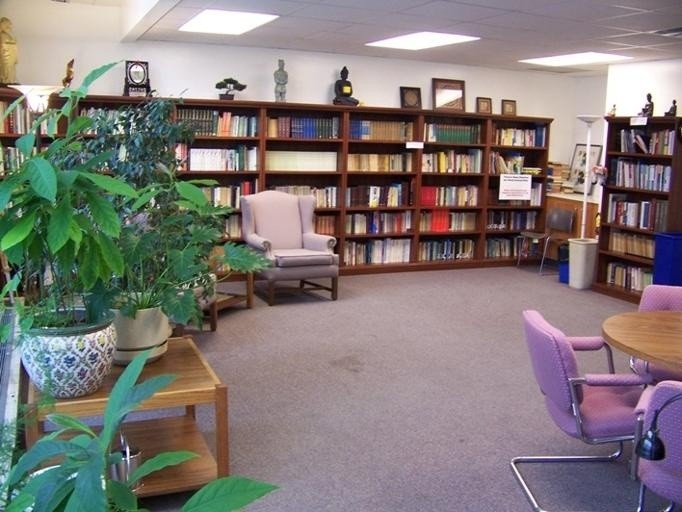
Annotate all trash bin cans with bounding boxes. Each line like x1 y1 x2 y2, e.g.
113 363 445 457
651 232 682 286
558 238 600 289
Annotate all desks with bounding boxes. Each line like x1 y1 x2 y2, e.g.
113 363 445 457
601 311 682 369
24 333 228 497
208 246 253 331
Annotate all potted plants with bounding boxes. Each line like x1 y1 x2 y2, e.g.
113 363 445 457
20 87 270 367
0 60 221 399
215 78 246 100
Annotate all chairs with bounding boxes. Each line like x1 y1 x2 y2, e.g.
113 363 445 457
628 285 682 383
239 190 340 309
510 309 656 512
126 273 218 336
637 380 682 511
516 207 577 274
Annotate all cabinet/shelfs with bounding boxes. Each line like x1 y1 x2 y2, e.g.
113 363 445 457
0 86 49 294
543 192 599 263
589 116 682 304
49 89 554 283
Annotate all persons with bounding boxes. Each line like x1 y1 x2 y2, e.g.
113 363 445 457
664 99 677 116
0 15 24 88
638 93 653 117
577 171 584 184
607 104 616 116
273 58 288 102
332 66 359 106
581 153 586 165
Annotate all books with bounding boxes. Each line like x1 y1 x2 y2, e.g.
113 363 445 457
200 178 258 210
174 110 340 171
79 107 140 171
312 213 337 235
277 186 336 207
226 215 243 238
343 121 545 267
600 125 677 297
0 101 57 176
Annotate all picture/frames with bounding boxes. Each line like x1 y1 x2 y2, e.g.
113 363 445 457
568 144 603 195
432 78 465 112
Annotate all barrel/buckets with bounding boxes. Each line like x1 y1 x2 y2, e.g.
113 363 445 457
559 262 572 284
108 433 144 493
